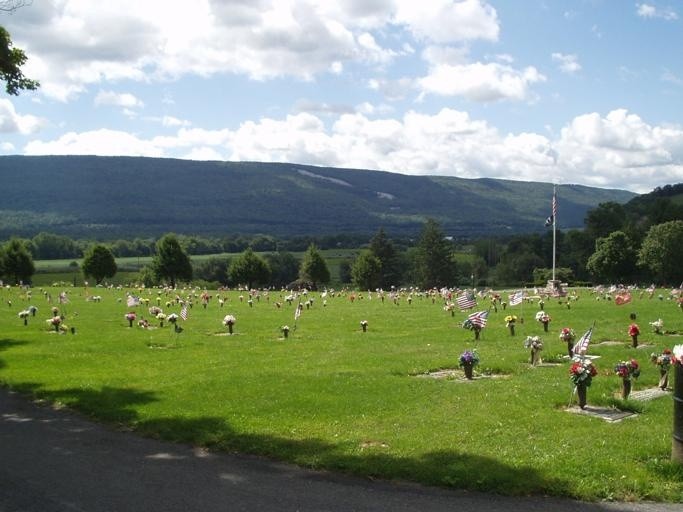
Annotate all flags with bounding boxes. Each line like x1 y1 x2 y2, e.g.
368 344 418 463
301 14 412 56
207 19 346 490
467 305 490 329
294 297 302 321
508 286 522 307
571 319 595 358
180 302 188 322
455 291 477 311
543 182 559 227
127 295 140 309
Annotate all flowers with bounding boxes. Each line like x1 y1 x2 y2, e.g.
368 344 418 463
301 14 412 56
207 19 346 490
569 349 675 389
457 311 642 368
18 296 237 331
